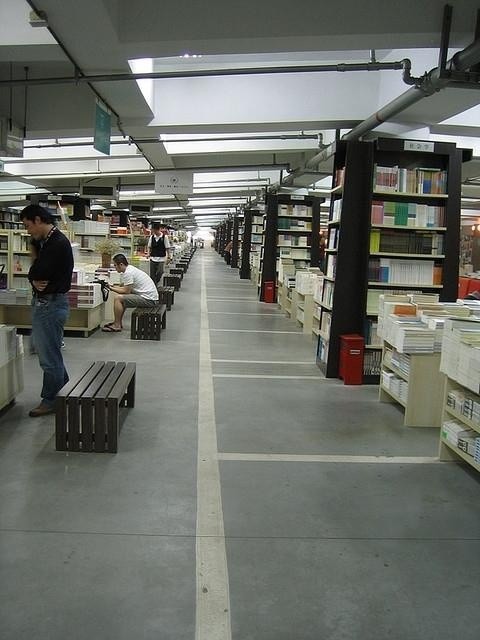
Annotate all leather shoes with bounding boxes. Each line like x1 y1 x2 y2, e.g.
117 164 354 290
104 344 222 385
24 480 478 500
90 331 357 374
29 399 58 418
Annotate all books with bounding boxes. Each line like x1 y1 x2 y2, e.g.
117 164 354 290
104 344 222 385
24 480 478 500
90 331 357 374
363 163 447 375
1 204 151 311
376 293 480 403
437 318 480 462
238 168 346 364
459 239 480 281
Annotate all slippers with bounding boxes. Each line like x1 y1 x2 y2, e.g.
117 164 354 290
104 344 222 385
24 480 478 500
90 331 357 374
102 322 123 332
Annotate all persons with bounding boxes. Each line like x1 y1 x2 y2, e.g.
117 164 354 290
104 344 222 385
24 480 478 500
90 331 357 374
17 204 73 417
101 253 159 331
145 223 171 283
224 241 233 265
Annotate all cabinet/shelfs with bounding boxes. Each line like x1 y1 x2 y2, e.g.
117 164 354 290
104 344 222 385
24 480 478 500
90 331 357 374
0 194 151 340
214 207 264 284
377 338 439 428
259 196 315 295
319 138 461 375
439 376 480 470
273 281 322 340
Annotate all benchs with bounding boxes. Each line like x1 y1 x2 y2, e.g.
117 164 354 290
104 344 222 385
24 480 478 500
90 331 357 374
131 244 196 340
55 359 138 453
